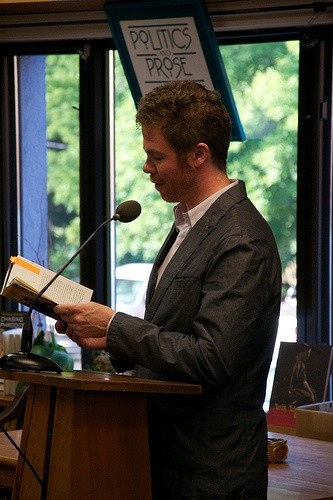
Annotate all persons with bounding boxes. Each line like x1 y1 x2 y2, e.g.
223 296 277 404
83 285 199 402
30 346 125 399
273 342 317 408
55 80 282 500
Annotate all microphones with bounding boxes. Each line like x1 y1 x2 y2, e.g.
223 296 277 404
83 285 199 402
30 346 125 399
0 200 142 374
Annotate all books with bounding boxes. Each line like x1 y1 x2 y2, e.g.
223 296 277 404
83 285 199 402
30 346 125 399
0 311 35 395
0 255 94 328
268 341 333 428
